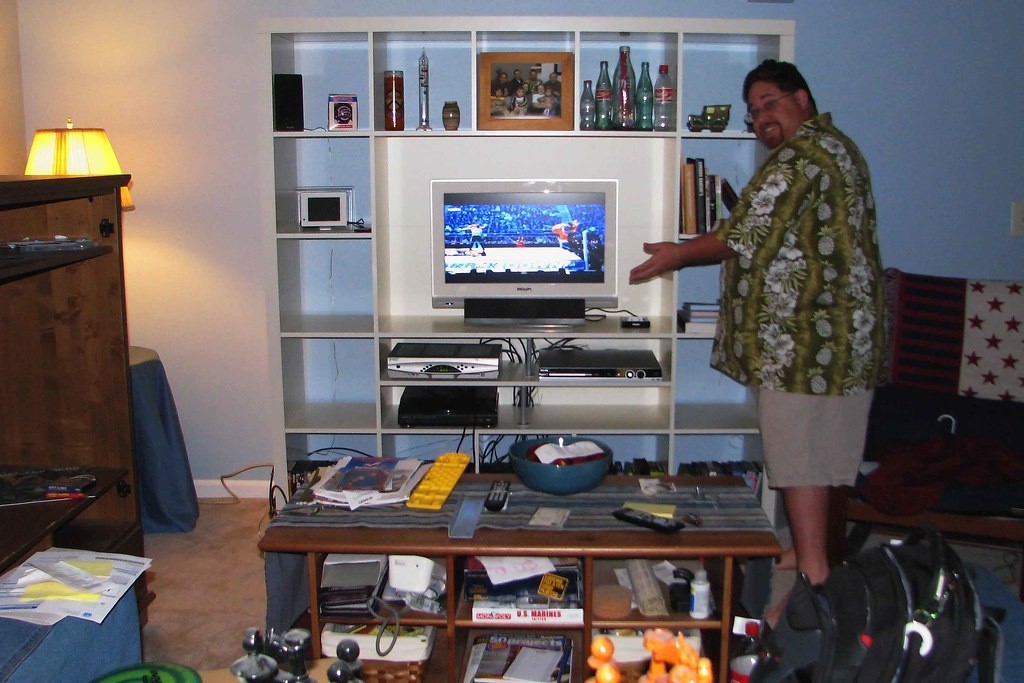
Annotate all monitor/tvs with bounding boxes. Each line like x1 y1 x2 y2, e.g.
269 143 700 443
300 193 347 230
430 180 618 328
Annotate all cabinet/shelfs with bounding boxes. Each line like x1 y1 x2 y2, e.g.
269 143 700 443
258 16 795 683
0 174 156 628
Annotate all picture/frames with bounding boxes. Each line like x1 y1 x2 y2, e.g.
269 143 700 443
478 52 574 131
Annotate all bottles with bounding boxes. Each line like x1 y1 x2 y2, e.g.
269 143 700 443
596 61 612 129
729 621 762 683
653 65 673 131
612 46 637 131
689 567 710 619
579 80 596 131
637 62 654 131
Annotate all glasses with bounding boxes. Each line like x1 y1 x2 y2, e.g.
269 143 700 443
750 92 793 117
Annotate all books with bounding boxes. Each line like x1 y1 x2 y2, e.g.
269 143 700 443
679 157 740 235
310 456 432 510
677 302 720 333
462 635 573 683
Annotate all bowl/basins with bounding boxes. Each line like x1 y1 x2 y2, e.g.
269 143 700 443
508 436 614 495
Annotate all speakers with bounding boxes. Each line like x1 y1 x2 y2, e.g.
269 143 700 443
274 74 305 131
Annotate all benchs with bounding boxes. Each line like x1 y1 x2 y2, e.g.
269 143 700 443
826 268 1024 599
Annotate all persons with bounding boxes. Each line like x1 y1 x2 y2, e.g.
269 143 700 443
441 202 604 273
490 69 561 117
629 59 884 625
332 105 353 125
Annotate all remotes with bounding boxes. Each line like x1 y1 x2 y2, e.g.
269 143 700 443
485 480 510 511
615 507 683 532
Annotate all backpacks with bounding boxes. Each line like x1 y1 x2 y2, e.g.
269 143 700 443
744 522 1004 683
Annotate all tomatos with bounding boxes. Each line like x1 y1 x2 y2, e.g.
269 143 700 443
523 442 603 464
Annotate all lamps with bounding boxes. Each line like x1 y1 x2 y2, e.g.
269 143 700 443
24 118 135 212
